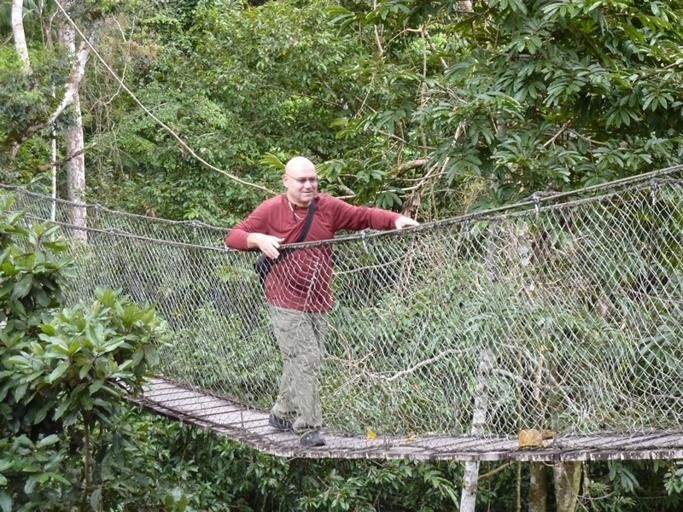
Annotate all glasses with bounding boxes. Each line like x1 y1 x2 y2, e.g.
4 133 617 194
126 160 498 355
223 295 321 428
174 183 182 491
289 174 318 183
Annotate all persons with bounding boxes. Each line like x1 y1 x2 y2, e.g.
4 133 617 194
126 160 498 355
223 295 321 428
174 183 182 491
224 156 421 448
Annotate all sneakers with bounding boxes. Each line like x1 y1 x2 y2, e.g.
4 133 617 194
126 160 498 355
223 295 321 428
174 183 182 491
268 411 301 436
299 426 325 447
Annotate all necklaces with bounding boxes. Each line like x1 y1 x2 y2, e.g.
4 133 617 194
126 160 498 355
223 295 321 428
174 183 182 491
288 202 309 220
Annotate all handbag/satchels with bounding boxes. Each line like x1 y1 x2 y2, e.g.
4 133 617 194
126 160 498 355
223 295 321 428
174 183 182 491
253 253 276 289
